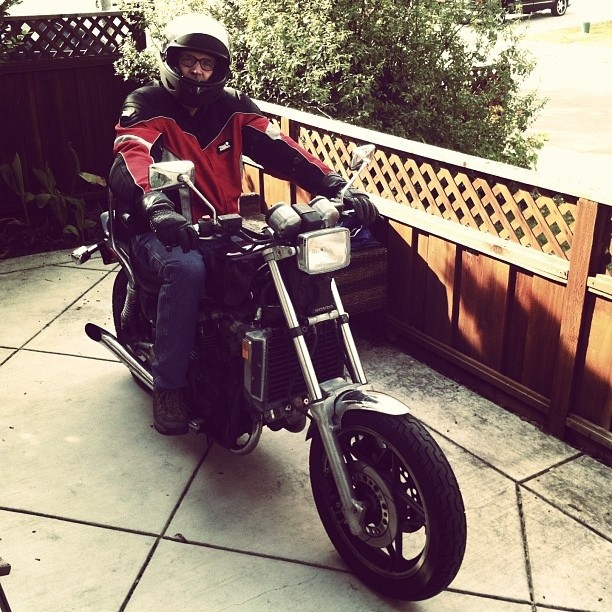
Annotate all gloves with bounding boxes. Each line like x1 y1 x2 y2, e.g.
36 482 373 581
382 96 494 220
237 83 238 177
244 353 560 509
320 171 380 225
135 190 199 254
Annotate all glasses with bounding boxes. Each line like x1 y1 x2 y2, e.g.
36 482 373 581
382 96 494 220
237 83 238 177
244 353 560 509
171 53 218 72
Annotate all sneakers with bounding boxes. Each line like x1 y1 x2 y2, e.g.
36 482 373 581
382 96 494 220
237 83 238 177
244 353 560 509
152 386 189 435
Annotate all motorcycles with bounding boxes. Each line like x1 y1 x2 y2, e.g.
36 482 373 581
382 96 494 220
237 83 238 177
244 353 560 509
71 143 466 600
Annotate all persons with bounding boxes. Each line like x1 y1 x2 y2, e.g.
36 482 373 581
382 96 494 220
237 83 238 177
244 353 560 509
106 12 380 437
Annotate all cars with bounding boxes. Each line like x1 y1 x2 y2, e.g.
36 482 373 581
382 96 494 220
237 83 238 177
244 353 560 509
474 0 570 25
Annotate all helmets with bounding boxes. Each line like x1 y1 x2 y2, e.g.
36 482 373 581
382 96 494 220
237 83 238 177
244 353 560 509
159 13 230 108
